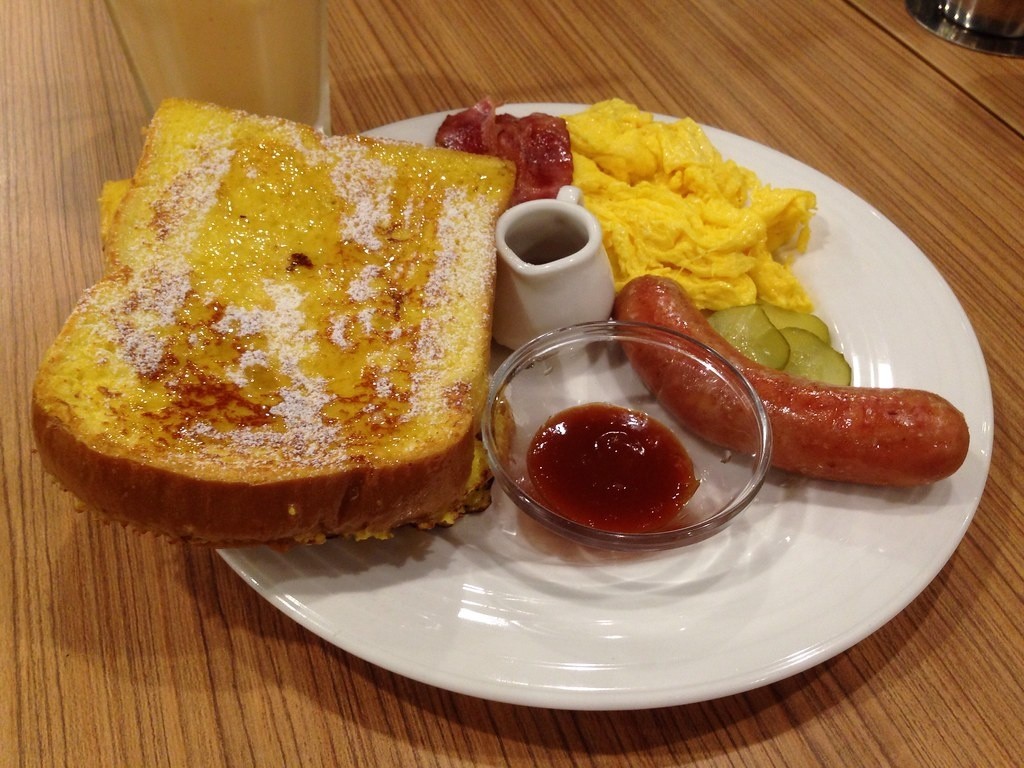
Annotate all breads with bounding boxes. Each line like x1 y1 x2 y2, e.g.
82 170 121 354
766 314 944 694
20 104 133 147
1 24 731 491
31 99 515 547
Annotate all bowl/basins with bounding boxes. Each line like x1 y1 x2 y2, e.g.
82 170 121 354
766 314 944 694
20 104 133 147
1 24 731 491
481 321 773 548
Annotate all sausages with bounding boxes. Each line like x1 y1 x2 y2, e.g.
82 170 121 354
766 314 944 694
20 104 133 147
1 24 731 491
614 276 971 486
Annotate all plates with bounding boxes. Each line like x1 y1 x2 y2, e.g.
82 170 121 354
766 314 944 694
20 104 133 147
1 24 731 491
216 103 993 711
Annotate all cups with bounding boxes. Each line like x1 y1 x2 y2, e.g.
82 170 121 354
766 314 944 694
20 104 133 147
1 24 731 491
493 186 614 356
